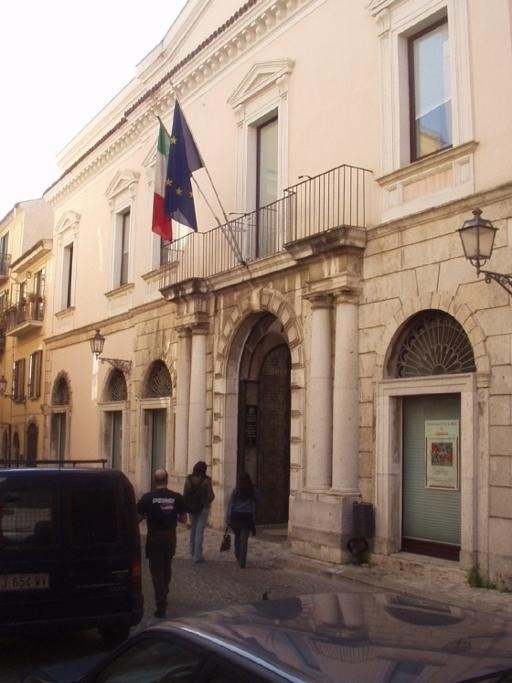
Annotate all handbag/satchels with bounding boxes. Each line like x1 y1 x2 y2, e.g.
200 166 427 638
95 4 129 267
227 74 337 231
220 534 231 552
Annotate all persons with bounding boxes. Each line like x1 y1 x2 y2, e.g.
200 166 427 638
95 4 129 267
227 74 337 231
182 460 218 564
227 471 260 570
134 466 189 621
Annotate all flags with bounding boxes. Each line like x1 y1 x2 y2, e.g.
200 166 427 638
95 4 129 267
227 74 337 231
151 121 178 244
162 106 206 234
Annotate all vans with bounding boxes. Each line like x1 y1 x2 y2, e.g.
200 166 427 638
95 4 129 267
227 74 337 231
0 457 144 650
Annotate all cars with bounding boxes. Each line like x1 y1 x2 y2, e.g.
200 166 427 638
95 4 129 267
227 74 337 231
74 590 512 683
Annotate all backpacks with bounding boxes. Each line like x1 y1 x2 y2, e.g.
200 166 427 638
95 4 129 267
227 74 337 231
184 476 206 514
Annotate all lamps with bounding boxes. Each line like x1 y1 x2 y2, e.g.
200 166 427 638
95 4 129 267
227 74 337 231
0 375 15 399
88 328 132 373
456 207 511 299
